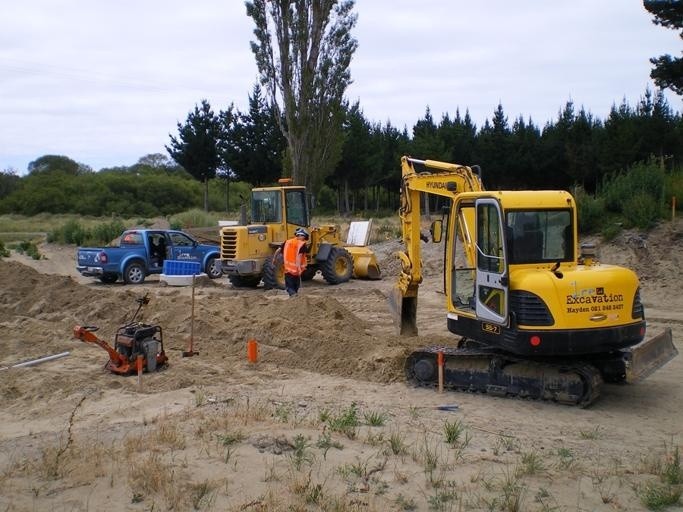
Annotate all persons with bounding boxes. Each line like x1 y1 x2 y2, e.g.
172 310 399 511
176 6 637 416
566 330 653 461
155 237 166 267
271 227 313 298
148 236 158 259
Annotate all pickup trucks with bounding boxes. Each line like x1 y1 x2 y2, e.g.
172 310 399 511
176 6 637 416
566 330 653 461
73 229 223 287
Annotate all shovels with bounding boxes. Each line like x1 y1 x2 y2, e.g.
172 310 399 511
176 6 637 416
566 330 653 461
183 273 199 357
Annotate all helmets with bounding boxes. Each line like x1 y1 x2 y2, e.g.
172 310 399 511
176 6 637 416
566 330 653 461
294 227 309 240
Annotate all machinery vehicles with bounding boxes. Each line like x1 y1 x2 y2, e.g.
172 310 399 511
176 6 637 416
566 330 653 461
219 177 382 294
388 155 680 409
